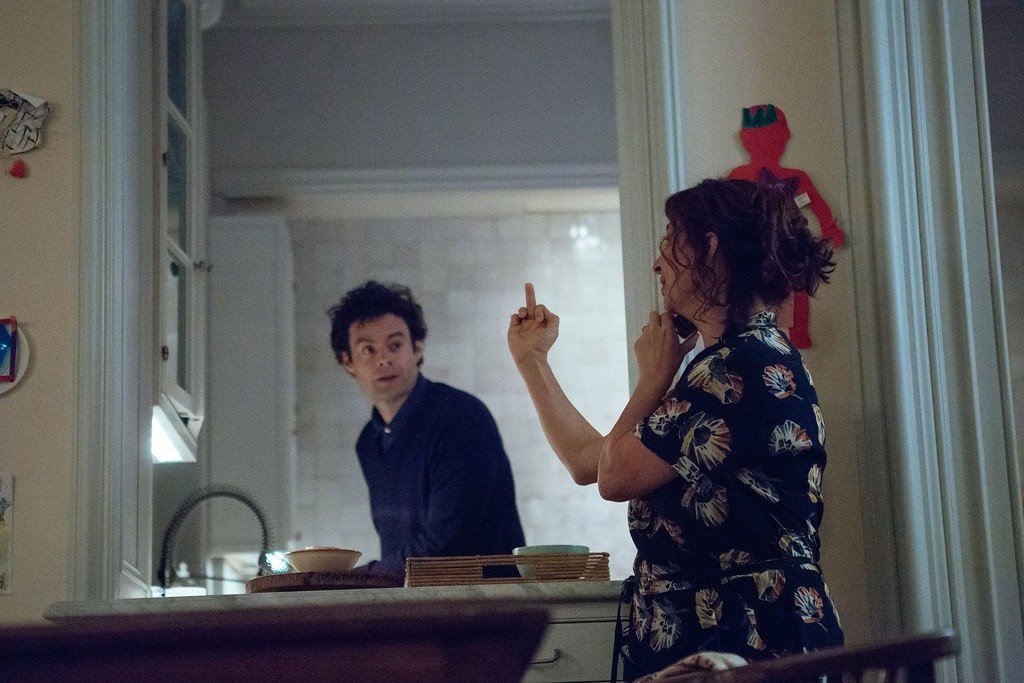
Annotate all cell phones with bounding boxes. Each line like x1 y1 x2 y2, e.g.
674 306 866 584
672 314 699 338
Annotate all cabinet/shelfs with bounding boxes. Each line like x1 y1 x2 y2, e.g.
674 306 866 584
151 0 207 465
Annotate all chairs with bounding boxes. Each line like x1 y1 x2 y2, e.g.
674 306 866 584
655 629 960 683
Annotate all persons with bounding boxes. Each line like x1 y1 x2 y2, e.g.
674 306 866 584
508 175 836 683
328 281 525 584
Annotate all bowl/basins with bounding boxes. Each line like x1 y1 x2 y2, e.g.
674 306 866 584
512 544 590 579
285 548 362 571
